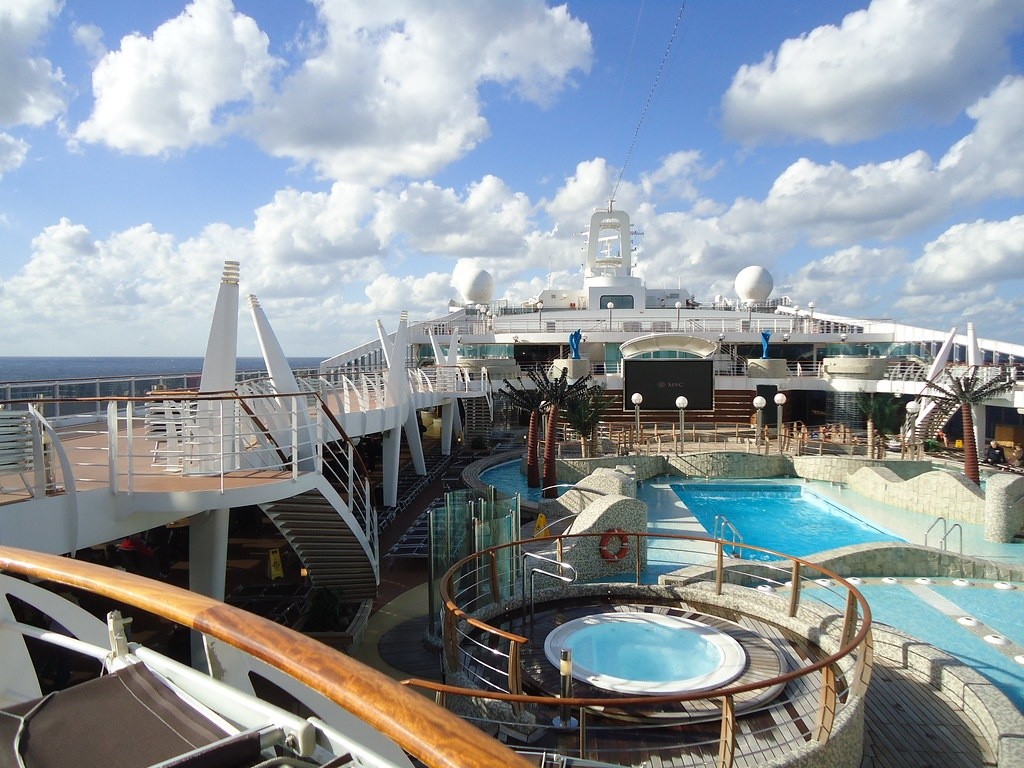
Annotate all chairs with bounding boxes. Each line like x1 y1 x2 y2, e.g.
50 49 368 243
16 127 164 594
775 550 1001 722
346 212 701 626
150 384 194 464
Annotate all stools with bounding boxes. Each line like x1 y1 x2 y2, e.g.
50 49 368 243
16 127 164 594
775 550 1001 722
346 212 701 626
955 439 963 449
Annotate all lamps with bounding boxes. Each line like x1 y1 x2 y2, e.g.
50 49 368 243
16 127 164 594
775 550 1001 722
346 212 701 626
581 332 588 343
718 334 726 341
512 335 519 343
783 334 790 341
840 334 848 342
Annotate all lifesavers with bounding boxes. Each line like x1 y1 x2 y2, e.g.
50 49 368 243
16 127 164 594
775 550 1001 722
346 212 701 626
599 528 631 563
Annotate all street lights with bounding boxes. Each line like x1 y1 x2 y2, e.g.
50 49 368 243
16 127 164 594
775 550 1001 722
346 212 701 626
748 301 753 330
752 395 766 452
607 301 614 331
631 391 643 447
807 302 816 334
905 401 920 460
675 395 688 454
674 302 682 331
475 304 497 333
774 393 786 453
537 302 544 332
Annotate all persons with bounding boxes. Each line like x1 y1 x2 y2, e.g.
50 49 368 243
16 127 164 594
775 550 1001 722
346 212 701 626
122 534 173 578
47 593 107 675
416 411 427 443
983 441 1008 465
1008 445 1024 465
